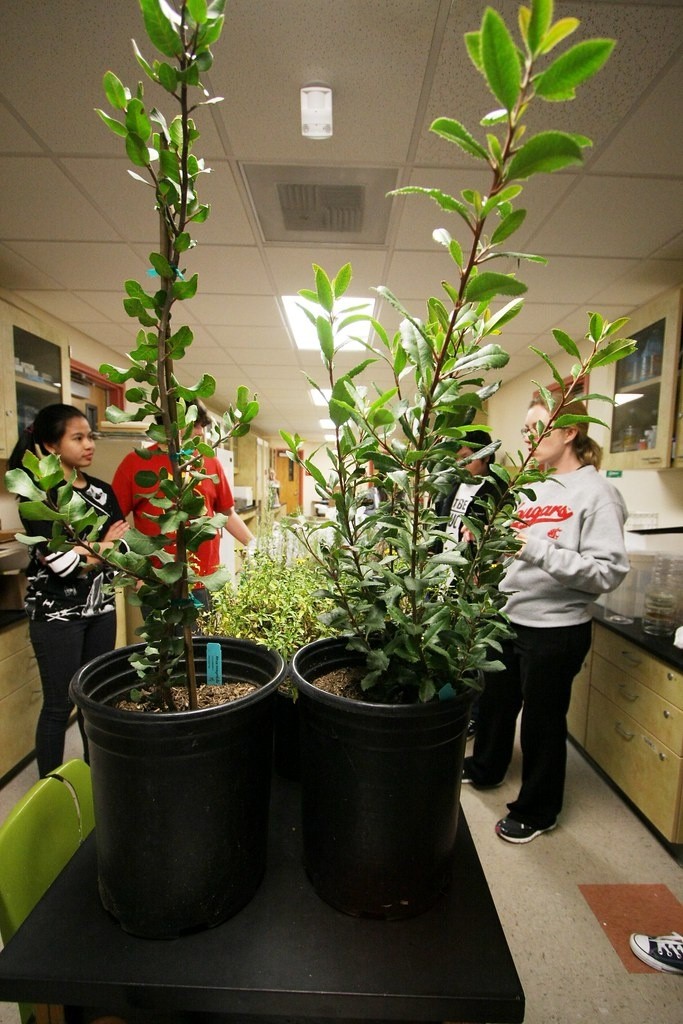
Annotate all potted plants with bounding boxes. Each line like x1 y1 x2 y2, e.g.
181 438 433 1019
7 0 298 928
293 3 627 917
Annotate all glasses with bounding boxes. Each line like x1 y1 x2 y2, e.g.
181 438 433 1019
521 425 568 436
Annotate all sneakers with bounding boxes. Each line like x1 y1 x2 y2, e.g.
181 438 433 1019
462 758 506 786
630 933 683 975
496 817 558 843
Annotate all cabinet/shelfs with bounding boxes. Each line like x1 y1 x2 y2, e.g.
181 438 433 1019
584 629 683 860
590 289 683 471
1 302 72 460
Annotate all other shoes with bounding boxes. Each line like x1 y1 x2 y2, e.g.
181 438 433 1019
467 720 478 740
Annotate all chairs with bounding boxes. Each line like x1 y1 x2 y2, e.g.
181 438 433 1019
0 759 132 1024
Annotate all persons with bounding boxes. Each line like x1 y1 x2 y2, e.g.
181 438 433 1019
111 397 253 637
629 931 683 975
6 405 131 778
434 394 629 844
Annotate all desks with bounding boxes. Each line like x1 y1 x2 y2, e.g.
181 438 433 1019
0 787 527 1024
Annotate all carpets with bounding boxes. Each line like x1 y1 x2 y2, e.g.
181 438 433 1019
577 882 683 972
